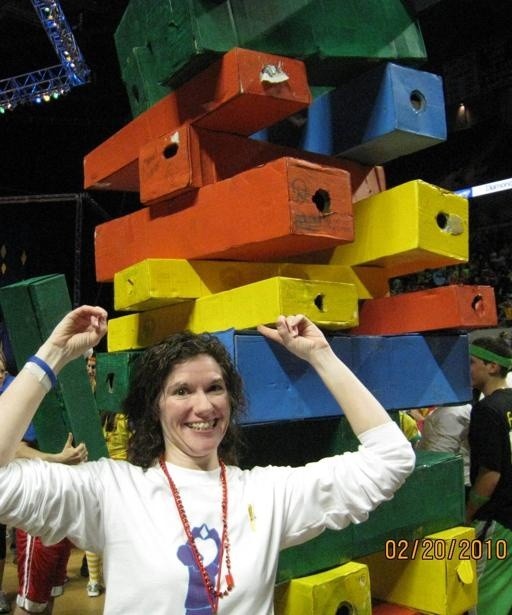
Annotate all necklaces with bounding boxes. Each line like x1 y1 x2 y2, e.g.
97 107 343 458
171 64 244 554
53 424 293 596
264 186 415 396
158 455 236 614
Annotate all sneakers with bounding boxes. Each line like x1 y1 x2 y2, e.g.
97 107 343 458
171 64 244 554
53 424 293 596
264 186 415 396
0 591 11 614
87 581 101 597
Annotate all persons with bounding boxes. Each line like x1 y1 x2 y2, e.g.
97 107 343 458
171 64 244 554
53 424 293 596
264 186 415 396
0 301 417 614
399 332 512 615
0 339 134 613
